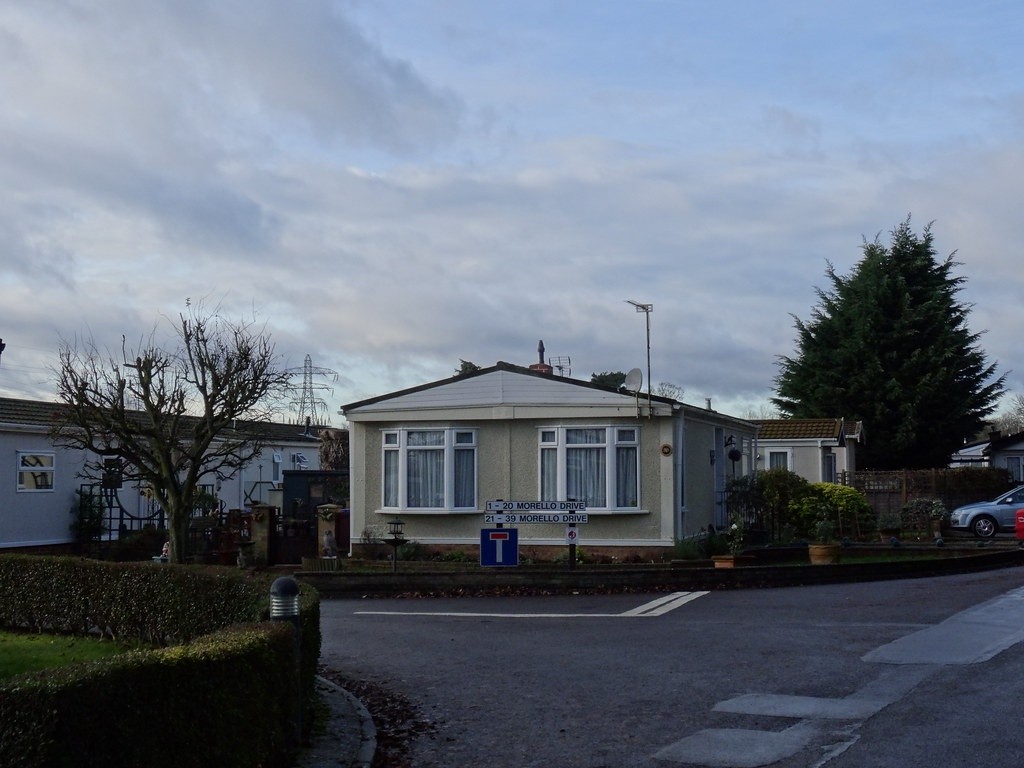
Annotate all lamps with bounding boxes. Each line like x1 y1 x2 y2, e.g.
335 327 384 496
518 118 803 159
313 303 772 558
269 577 302 622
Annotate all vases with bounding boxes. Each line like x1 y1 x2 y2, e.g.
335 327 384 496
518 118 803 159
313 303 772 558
711 554 749 567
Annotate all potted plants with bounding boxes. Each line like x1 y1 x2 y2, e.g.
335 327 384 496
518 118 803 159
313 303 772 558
929 500 952 537
808 518 844 564
877 512 903 543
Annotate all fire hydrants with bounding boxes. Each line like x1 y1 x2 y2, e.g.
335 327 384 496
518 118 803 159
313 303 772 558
1015 509 1024 541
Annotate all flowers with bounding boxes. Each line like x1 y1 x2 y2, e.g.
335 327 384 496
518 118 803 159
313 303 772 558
720 511 747 557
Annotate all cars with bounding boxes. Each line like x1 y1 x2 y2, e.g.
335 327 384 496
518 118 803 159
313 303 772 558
950 485 1024 539
